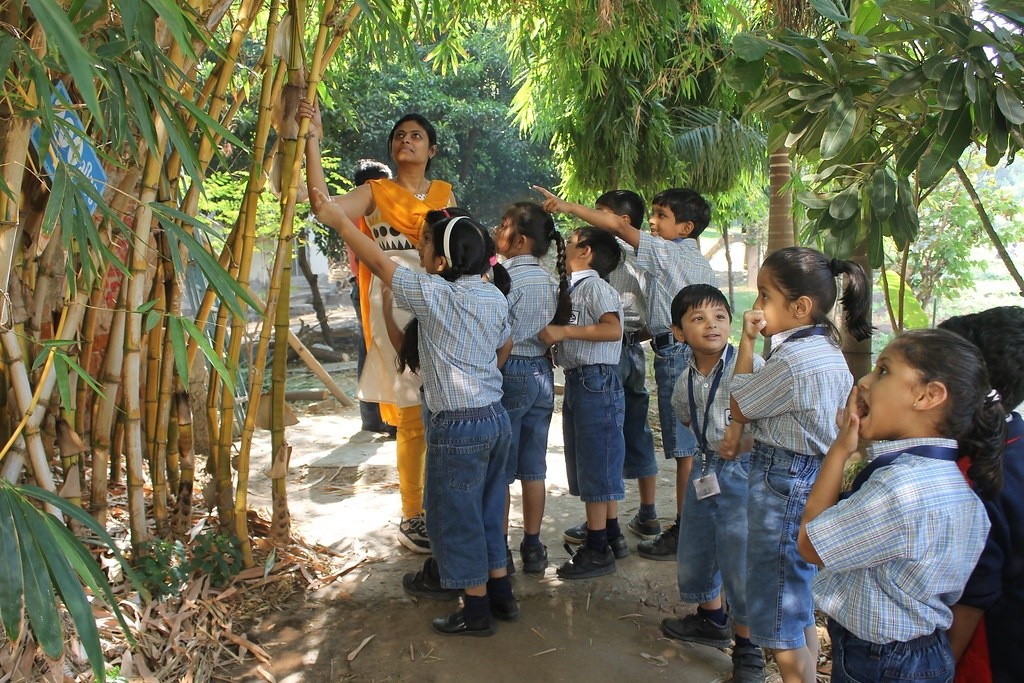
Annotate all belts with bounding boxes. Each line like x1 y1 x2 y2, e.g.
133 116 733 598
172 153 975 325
623 326 652 345
650 332 678 352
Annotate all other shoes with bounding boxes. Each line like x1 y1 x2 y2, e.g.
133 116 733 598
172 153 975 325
362 421 388 431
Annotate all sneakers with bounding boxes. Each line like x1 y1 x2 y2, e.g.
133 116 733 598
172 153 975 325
556 543 617 578
486 574 519 618
563 521 587 542
661 613 731 647
430 594 495 637
397 513 432 553
520 542 548 573
627 512 662 539
503 550 515 573
732 645 765 683
637 524 680 560
609 540 630 560
402 557 459 598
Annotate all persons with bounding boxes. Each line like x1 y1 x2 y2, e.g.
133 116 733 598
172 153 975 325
381 206 489 596
799 327 1006 683
346 159 396 432
490 200 573 574
937 306 1024 683
314 188 520 636
729 246 874 683
562 189 661 540
532 185 717 560
295 94 457 554
539 226 629 577
660 282 766 683
